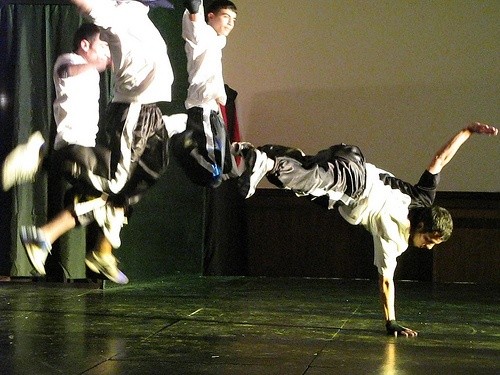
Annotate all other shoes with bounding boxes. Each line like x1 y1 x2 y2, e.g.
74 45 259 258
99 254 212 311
162 113 188 138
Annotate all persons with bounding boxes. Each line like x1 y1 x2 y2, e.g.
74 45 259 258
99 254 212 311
230 122 499 336
17 24 129 286
162 0 238 186
3 0 175 197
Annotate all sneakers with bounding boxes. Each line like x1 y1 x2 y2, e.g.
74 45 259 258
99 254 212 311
84 251 129 284
93 202 125 249
228 142 255 178
235 147 268 200
2 131 45 191
20 225 52 275
